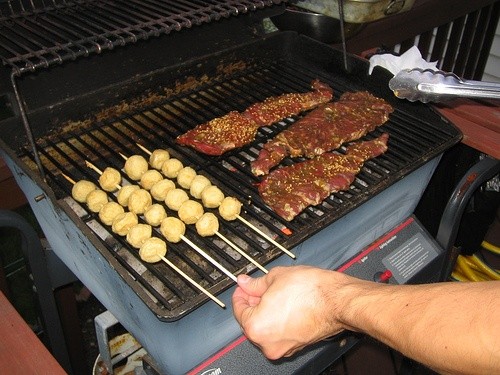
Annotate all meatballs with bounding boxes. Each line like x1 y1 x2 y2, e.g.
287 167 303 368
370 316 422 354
150 149 241 221
97 167 185 243
72 180 166 262
124 155 219 236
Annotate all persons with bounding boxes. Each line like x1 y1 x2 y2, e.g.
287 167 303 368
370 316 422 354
231 264 500 375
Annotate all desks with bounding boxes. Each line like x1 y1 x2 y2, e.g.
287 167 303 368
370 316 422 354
271 0 499 81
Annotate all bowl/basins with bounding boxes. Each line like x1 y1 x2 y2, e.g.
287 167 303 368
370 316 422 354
269 6 368 43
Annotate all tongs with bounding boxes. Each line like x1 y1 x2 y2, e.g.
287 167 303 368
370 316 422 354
388 68 499 103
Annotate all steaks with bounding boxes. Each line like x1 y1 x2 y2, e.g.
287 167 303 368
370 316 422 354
176 77 334 155
250 90 394 177
258 134 389 222
